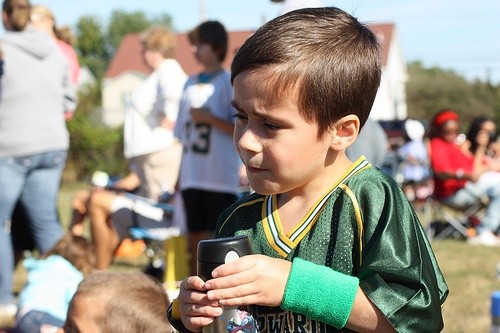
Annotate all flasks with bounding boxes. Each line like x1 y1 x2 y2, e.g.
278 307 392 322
196 235 258 333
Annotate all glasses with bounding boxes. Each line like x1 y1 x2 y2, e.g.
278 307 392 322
442 128 464 133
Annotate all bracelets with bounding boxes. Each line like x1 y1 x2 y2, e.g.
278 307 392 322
456 168 464 178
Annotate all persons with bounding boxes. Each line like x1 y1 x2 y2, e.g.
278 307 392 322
167 6 449 333
17 233 96 333
430 108 500 245
0 0 79 316
57 272 175 333
69 25 189 281
398 118 432 200
30 5 80 121
173 21 243 276
